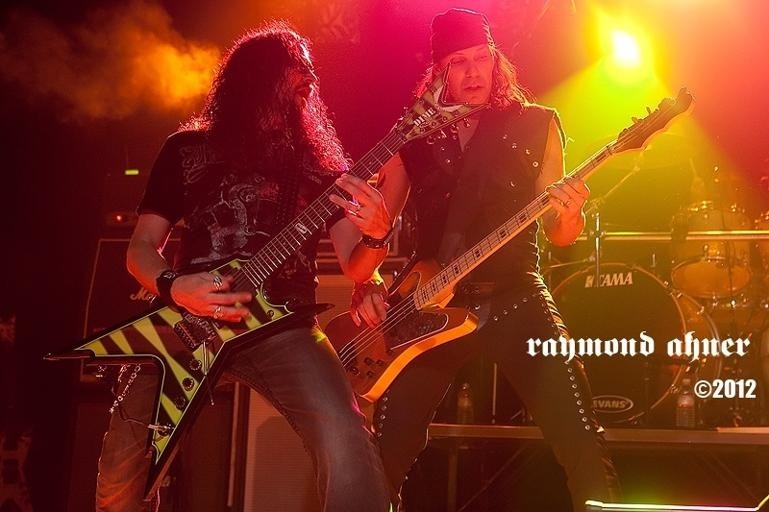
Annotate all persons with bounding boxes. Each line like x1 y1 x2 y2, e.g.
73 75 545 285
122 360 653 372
349 6 622 512
94 29 390 512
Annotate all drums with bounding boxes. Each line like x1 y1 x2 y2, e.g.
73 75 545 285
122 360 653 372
668 199 752 300
550 261 721 426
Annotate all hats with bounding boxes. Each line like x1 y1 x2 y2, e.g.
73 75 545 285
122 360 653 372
430 7 495 55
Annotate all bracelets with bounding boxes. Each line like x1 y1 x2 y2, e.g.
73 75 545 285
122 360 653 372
361 227 394 249
155 269 183 309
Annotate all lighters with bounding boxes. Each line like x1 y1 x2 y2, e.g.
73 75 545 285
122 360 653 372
565 198 572 206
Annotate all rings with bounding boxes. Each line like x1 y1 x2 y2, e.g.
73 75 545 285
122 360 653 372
213 275 222 292
212 305 224 322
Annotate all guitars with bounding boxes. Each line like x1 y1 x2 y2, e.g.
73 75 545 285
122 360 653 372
325 85 693 405
43 62 488 505
